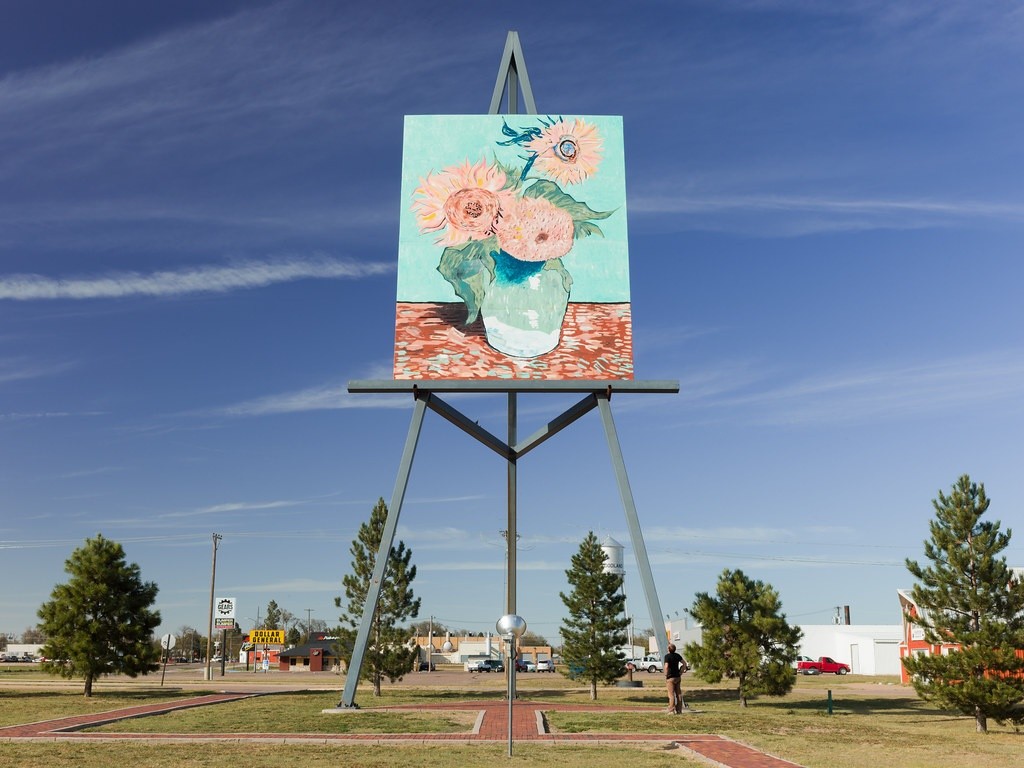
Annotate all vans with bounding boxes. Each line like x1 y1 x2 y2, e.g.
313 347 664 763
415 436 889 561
791 655 815 669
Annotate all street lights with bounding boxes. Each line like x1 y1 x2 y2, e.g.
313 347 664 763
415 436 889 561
244 616 257 673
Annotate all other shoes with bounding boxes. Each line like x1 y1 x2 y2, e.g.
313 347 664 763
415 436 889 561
676 710 682 714
668 711 675 715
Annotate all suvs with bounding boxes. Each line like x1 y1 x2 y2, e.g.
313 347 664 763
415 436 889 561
537 659 555 673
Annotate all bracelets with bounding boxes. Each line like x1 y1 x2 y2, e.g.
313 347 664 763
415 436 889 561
665 675 668 676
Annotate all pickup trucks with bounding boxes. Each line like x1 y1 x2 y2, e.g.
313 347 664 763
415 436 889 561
797 656 851 675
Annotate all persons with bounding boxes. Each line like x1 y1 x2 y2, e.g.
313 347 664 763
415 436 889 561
663 644 687 715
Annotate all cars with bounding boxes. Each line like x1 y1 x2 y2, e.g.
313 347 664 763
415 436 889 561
625 655 691 674
468 659 491 673
419 662 436 671
487 659 537 672
